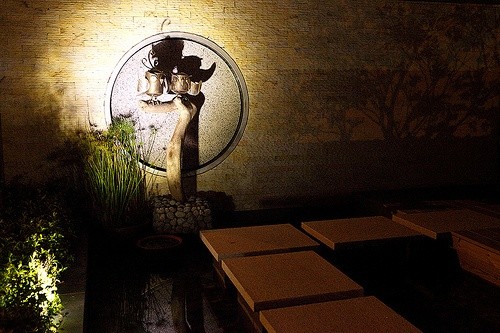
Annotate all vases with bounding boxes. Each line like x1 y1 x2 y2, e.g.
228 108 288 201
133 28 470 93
134 232 183 268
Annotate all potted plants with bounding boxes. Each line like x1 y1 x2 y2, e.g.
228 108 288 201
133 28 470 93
63 109 168 257
